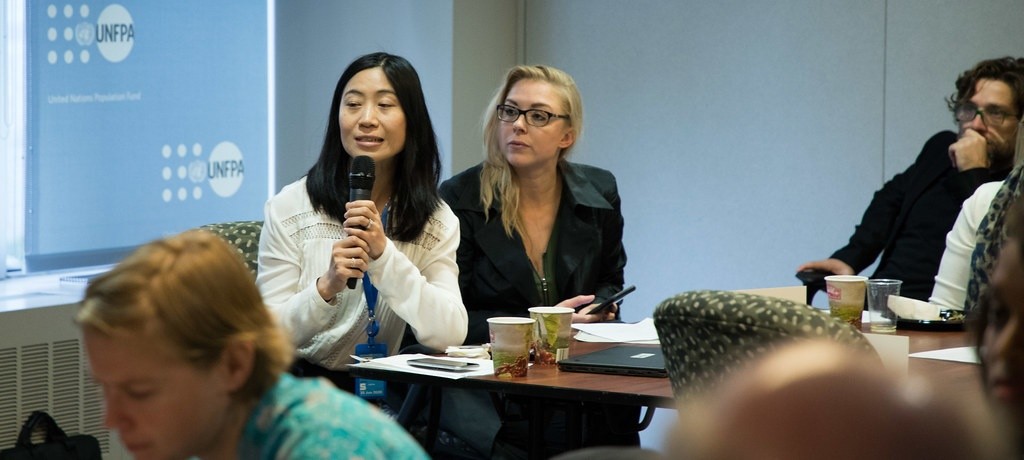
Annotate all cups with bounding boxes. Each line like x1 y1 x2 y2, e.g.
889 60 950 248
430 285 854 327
487 317 535 378
528 307 575 364
867 280 903 331
823 275 868 329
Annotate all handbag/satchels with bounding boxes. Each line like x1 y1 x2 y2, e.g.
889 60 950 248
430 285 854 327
0 409 103 460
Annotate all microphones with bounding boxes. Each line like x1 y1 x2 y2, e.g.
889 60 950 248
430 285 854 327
345 155 375 289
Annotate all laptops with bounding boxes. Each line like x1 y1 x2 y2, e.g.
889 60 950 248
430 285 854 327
558 345 670 379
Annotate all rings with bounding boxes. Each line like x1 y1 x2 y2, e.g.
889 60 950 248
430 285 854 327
351 258 356 268
365 218 373 229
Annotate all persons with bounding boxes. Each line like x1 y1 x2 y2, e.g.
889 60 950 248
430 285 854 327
72 227 434 460
254 51 469 460
436 63 641 460
658 159 1024 460
796 55 1024 330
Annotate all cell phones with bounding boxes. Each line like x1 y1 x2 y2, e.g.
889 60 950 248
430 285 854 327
584 286 637 315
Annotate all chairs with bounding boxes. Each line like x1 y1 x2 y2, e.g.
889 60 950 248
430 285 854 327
208 223 263 281
652 286 883 417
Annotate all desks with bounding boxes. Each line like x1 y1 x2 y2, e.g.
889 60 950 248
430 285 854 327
351 319 985 460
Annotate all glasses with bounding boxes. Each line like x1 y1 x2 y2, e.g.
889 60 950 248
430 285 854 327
495 103 570 128
953 102 1018 126
